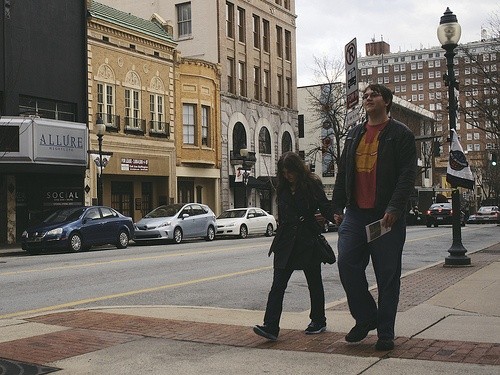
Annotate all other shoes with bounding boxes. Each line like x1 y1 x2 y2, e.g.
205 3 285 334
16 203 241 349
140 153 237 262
305 320 327 334
375 338 393 350
344 323 376 342
253 324 280 340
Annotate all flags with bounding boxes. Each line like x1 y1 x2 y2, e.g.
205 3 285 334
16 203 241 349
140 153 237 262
446 128 474 189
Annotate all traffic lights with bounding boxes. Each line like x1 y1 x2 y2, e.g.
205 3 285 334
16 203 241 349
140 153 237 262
491 152 497 166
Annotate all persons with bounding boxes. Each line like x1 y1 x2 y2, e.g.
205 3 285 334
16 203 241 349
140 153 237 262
252 152 340 340
332 83 417 352
413 206 419 224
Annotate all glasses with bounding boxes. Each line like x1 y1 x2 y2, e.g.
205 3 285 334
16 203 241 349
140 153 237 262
362 92 382 100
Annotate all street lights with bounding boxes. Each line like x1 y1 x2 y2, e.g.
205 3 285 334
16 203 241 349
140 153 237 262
437 6 476 268
239 143 248 207
93 117 106 206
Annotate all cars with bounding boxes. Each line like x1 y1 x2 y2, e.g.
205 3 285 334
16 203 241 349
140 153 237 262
315 208 341 233
131 202 218 245
426 202 466 227
466 214 477 224
21 205 136 254
214 207 278 239
476 205 500 224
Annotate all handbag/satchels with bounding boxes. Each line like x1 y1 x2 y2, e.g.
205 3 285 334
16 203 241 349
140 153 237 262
316 234 337 265
